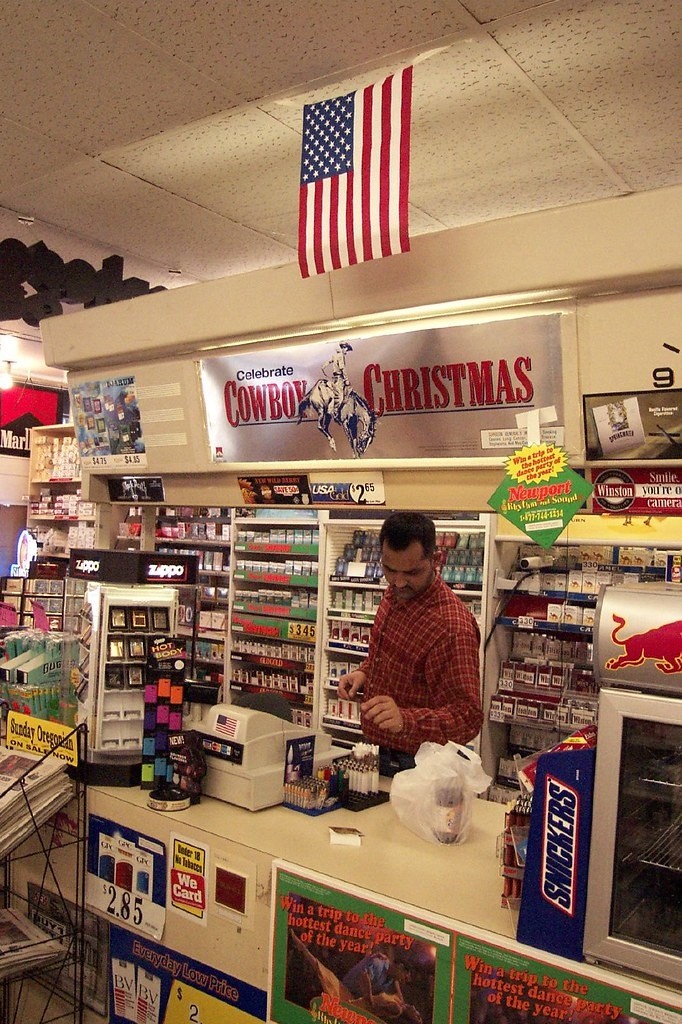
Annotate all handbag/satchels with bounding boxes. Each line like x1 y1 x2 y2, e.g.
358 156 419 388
390 740 494 847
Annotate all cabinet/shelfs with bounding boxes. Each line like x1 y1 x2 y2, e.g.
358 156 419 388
27 425 125 551
109 501 237 706
494 535 682 796
323 520 491 765
0 723 90 1023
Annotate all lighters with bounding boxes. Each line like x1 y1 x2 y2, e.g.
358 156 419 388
283 764 349 811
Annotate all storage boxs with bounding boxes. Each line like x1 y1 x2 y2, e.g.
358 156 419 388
1 436 96 731
128 507 487 729
491 546 681 785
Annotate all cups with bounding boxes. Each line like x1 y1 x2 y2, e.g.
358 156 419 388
116 861 133 892
99 855 115 883
136 871 149 893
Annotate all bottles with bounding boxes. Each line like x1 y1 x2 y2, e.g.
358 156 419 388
434 776 463 843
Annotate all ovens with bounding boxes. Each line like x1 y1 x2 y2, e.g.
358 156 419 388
582 686 682 989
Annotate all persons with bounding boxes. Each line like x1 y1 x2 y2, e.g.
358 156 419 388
288 927 423 1024
336 511 484 780
